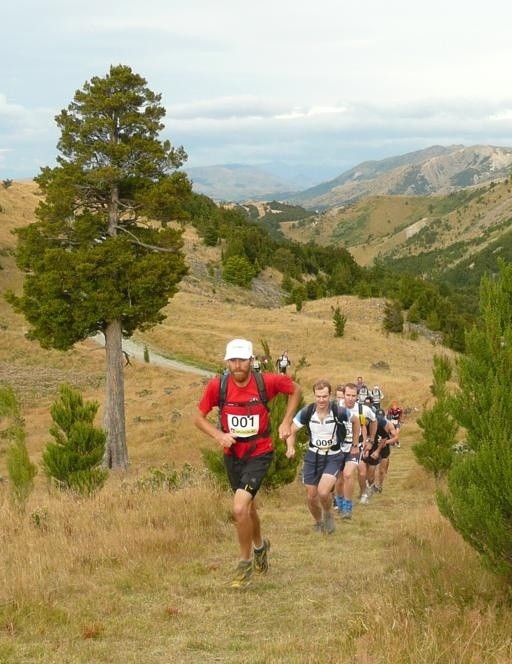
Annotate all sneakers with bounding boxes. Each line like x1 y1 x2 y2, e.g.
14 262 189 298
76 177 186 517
323 511 334 534
331 499 339 510
359 479 382 504
340 500 352 519
313 521 323 531
394 440 400 447
252 537 270 575
336 496 344 511
231 558 253 589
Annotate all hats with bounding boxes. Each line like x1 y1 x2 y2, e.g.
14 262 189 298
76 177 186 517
224 338 253 361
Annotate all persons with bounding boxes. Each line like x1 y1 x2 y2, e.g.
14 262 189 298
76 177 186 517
276 351 290 374
286 377 405 533
193 338 303 591
